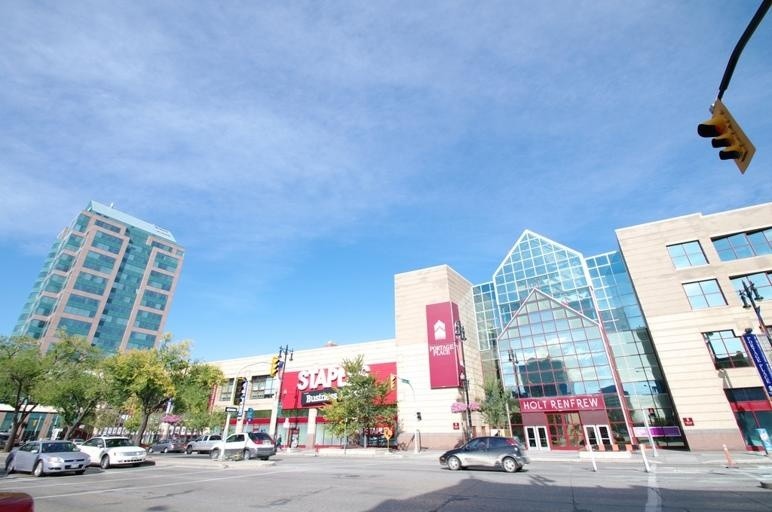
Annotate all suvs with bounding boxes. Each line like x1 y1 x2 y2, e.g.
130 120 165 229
438 434 532 472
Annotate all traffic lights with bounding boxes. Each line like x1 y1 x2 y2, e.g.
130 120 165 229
390 372 396 390
695 98 755 176
230 376 245 408
271 355 280 377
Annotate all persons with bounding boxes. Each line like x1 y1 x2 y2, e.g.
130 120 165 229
274 433 283 452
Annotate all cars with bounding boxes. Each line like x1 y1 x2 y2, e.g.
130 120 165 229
1 428 276 478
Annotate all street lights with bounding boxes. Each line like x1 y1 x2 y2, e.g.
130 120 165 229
274 344 295 440
737 277 772 348
507 346 521 398
454 319 473 442
